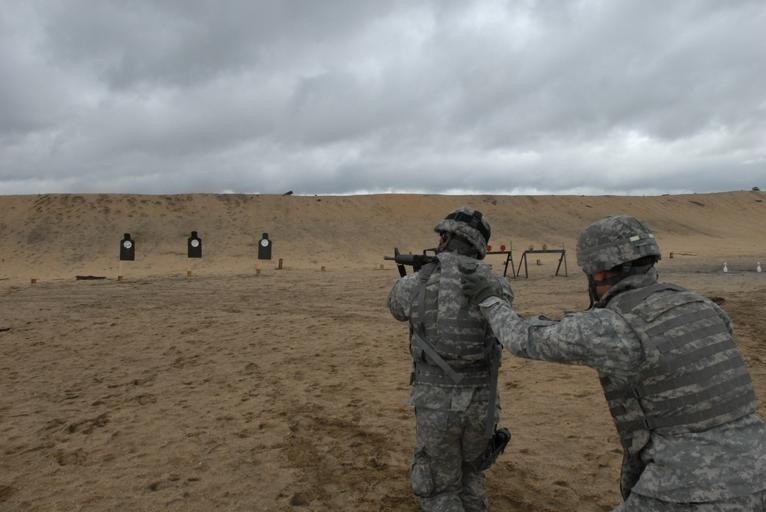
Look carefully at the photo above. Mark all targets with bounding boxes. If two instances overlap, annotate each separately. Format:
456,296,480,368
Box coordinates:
121,232,135,261
258,232,272,259
188,231,202,258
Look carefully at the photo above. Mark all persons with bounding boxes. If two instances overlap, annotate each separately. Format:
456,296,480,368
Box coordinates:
385,207,515,512
461,215,766,512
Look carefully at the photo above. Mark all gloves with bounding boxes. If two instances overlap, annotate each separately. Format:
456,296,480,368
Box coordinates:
461,272,500,305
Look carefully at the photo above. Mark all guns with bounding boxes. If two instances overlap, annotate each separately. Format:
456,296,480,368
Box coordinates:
383,246,437,277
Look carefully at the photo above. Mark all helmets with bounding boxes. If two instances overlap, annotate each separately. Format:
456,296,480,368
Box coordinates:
433,206,493,261
576,214,662,275
